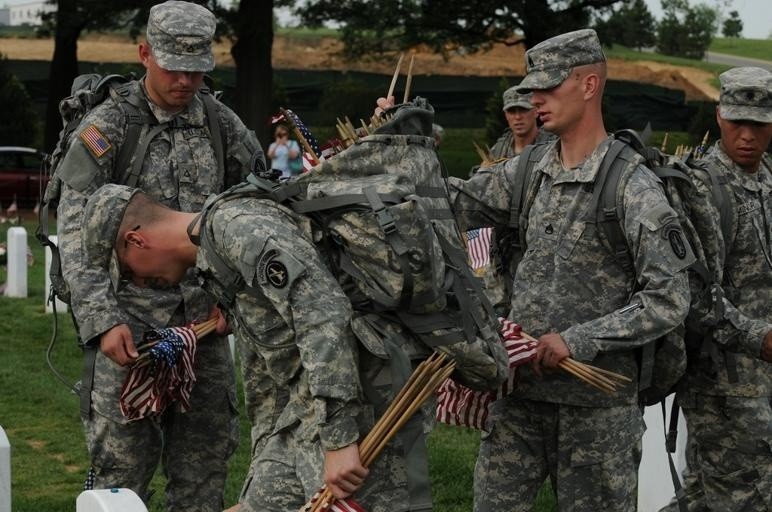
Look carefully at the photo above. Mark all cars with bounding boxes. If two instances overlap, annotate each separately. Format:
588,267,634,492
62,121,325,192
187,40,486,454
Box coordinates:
0,147,62,208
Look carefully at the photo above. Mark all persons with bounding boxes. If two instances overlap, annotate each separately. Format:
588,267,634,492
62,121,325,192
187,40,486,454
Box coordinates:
72,175,433,511
265,127,304,169
366,29,691,511
658,66,769,512
48,2,266,511
475,84,571,313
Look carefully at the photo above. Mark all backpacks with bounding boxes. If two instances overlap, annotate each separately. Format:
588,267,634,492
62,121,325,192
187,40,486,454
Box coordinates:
43,71,227,304
202,133,510,391
511,128,726,406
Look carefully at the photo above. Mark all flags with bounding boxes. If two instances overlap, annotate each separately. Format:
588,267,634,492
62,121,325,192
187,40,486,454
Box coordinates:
316,139,347,160
117,318,207,423
454,222,505,273
435,313,555,433
279,109,327,172
300,483,367,512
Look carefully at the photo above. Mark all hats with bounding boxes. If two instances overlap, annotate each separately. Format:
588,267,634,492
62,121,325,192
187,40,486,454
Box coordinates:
81,184,144,293
503,28,607,111
146,0,217,73
718,66,771,124
